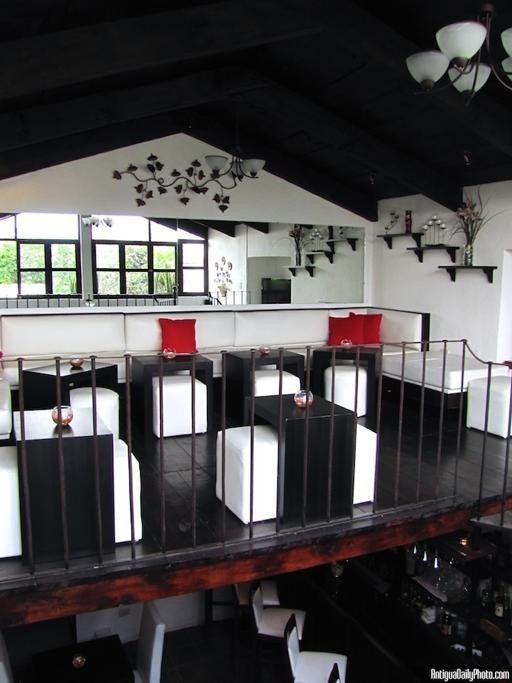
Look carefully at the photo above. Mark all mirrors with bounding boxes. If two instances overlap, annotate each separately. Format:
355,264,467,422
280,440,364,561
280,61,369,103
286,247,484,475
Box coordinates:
1,210,365,304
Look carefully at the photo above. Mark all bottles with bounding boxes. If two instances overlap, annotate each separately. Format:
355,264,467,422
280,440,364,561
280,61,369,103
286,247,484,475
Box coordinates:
408,542,511,635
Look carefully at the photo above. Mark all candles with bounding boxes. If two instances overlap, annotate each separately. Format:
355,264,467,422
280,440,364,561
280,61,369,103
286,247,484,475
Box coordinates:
309,228,323,239
423,214,445,229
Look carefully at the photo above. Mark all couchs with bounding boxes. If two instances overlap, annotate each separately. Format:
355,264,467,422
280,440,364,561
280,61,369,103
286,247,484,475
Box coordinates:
0,305,431,443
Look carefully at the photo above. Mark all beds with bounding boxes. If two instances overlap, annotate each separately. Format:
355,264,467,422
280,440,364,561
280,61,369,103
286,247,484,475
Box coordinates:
243,393,358,529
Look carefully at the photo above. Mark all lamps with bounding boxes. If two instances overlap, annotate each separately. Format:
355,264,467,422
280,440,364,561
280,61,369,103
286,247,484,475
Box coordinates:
405,3,512,110
204,93,267,182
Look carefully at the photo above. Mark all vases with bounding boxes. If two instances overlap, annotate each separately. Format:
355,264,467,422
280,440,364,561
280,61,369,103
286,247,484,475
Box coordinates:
296,249,300,266
464,244,472,266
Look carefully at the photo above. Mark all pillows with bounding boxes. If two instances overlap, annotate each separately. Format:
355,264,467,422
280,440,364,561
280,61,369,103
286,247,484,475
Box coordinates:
157,318,199,354
329,312,383,346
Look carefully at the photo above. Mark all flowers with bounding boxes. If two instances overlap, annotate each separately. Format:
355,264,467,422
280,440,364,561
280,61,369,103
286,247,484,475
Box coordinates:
448,182,506,247
286,224,311,252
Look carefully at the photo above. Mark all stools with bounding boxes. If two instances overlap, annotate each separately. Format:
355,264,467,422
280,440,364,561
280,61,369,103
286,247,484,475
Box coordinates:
324,364,366,418
250,369,301,397
466,375,511,438
215,422,377,525
152,375,208,438
1,439,144,559
69,388,119,439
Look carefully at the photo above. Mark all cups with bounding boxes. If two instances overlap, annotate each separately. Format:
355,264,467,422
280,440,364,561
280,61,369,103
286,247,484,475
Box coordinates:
293,389,314,408
162,347,177,359
259,344,271,355
70,356,84,368
70,654,87,671
340,338,353,350
51,404,75,425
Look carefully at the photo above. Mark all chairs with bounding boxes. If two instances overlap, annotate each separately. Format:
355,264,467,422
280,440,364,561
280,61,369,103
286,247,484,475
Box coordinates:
132,576,351,683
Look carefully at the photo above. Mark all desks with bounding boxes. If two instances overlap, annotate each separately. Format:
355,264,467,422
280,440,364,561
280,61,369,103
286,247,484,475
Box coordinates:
21,633,137,683
